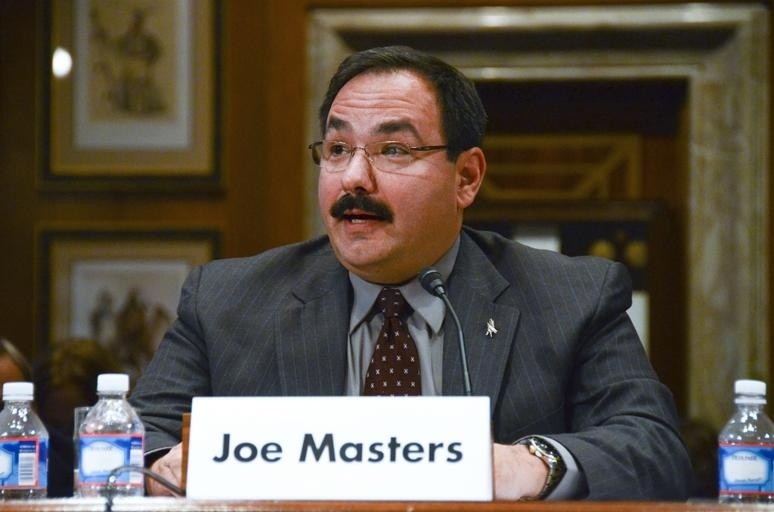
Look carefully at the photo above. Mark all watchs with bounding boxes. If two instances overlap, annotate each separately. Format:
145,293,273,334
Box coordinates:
518,437,566,502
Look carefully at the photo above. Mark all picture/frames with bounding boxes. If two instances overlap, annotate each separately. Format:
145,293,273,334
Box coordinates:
32,0,232,197
36,224,221,436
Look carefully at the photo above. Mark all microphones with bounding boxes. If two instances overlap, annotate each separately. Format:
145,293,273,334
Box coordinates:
419,265,473,397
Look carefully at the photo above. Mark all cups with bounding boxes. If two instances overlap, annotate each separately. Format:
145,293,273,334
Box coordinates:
69,404,92,511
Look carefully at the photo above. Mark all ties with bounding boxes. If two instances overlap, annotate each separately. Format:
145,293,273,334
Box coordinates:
361,289,423,397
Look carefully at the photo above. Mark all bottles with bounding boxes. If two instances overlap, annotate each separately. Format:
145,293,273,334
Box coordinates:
716,379,773,512
76,371,145,511
0,380,52,511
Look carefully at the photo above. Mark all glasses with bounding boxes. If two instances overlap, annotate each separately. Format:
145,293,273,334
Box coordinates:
308,138,446,174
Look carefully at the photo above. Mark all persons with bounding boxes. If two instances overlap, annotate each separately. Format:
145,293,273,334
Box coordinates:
0,337,75,499
125,43,699,504
